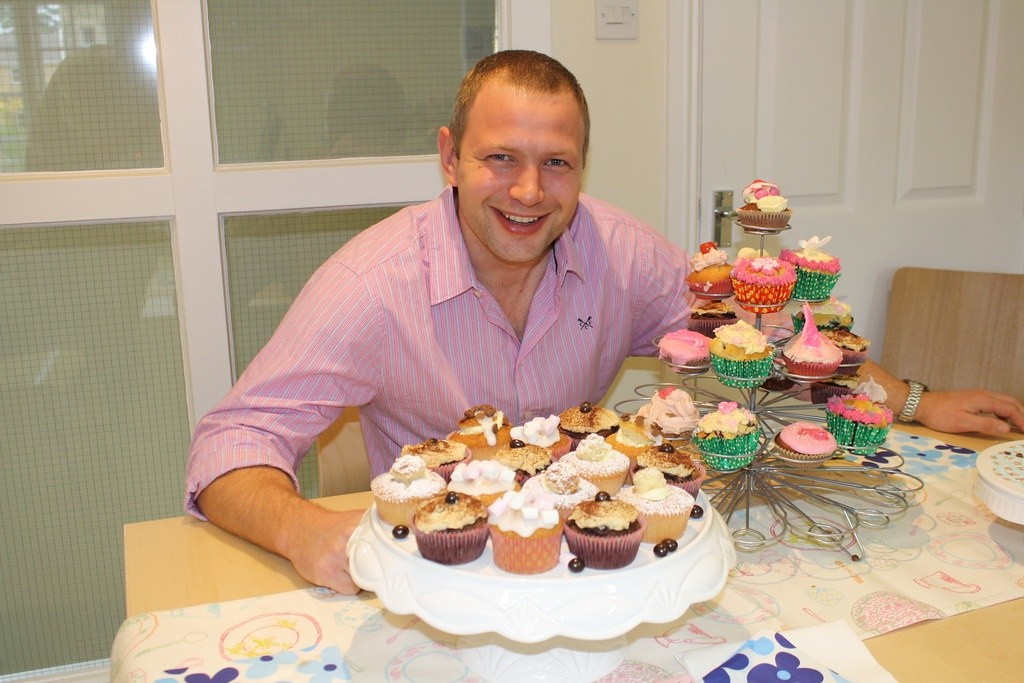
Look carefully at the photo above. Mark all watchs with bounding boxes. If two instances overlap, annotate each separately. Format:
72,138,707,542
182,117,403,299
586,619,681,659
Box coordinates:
898,379,929,424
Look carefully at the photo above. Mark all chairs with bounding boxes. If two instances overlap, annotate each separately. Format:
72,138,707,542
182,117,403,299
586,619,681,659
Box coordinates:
878,266,1024,409
318,400,373,499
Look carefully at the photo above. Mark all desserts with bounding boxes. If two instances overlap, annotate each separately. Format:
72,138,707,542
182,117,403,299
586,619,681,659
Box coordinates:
636,179,893,472
370,402,705,575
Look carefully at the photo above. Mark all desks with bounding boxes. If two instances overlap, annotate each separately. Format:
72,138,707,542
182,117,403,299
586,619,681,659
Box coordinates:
121,422,1024,682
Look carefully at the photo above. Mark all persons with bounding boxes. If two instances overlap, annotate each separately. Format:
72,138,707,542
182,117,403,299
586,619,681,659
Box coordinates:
183,50,1024,595
